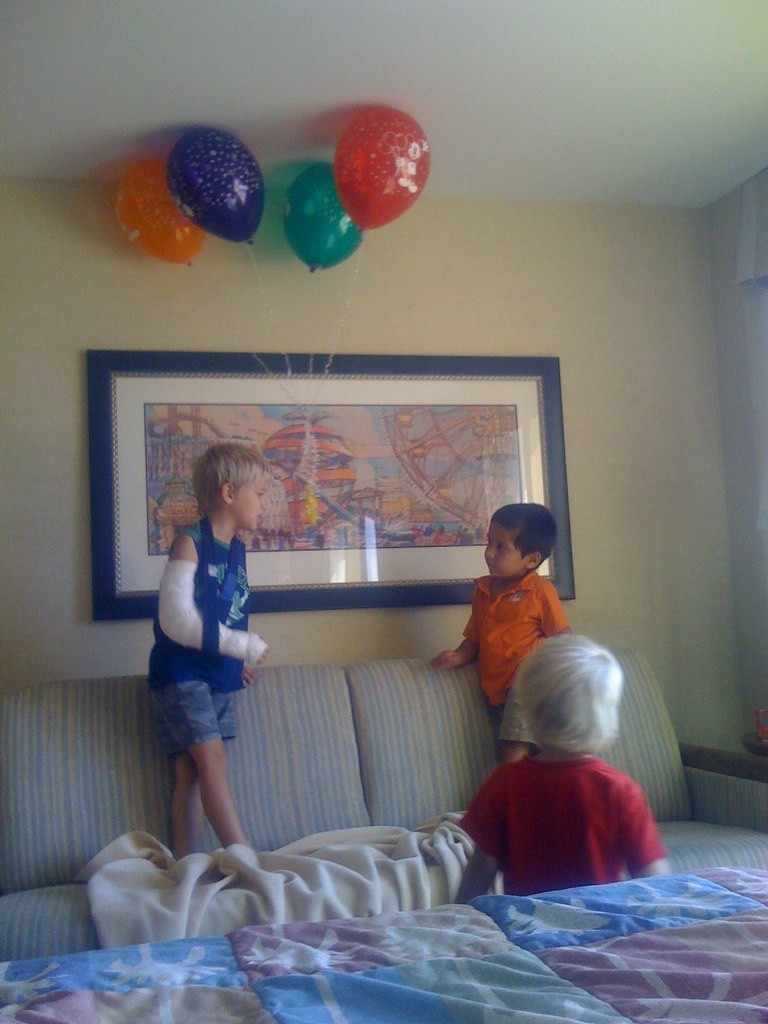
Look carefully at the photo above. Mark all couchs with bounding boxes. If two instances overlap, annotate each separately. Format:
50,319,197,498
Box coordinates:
0,645,768,962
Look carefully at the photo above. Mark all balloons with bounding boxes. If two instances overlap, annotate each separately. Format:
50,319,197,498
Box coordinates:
112,107,433,272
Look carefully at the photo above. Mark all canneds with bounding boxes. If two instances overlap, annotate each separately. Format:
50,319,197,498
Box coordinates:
755,708,768,741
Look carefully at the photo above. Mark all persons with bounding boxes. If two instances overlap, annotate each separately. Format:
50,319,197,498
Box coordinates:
453,633,676,905
431,503,571,764
148,443,274,857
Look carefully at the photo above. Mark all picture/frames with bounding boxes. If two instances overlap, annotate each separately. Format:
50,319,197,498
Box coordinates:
89,349,576,618
